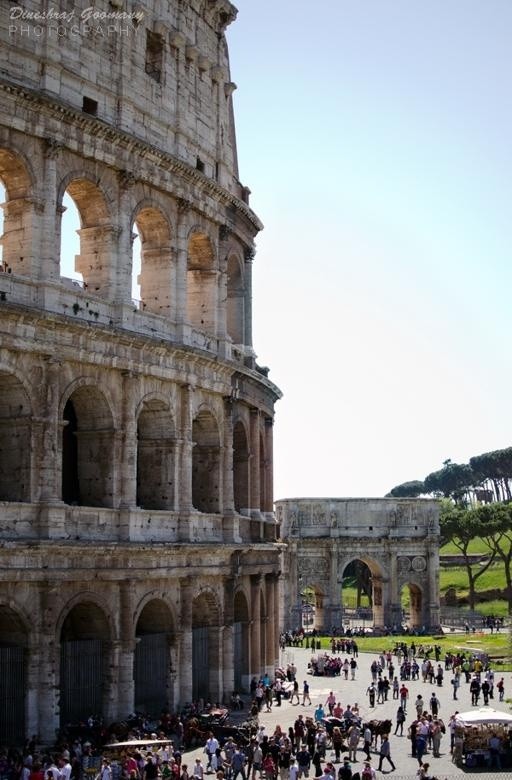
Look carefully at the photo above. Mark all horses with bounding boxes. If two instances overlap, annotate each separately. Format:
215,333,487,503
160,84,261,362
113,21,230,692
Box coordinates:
368,719,393,752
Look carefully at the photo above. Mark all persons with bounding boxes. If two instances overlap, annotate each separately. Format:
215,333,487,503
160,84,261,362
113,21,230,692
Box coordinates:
0,614,512,780
